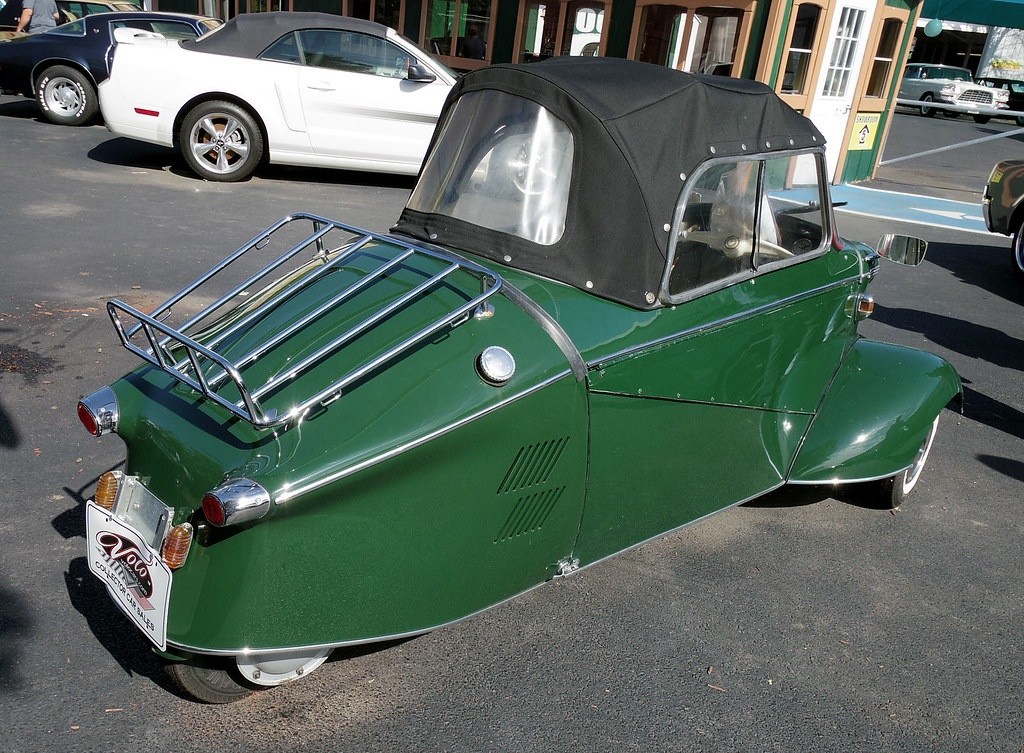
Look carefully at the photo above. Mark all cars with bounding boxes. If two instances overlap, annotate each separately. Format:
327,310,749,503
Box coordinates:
0,10,225,129
896,65,1013,125
91,10,567,206
0,1,163,33
81,51,971,706
982,159,1024,304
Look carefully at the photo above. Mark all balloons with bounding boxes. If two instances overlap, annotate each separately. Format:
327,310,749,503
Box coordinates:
924,19,942,37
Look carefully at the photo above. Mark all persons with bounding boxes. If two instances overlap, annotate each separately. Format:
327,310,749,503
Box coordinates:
17,0,59,34
458,23,483,59
908,67,927,78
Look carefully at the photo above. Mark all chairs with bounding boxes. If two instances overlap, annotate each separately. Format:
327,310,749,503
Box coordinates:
306,41,324,67
